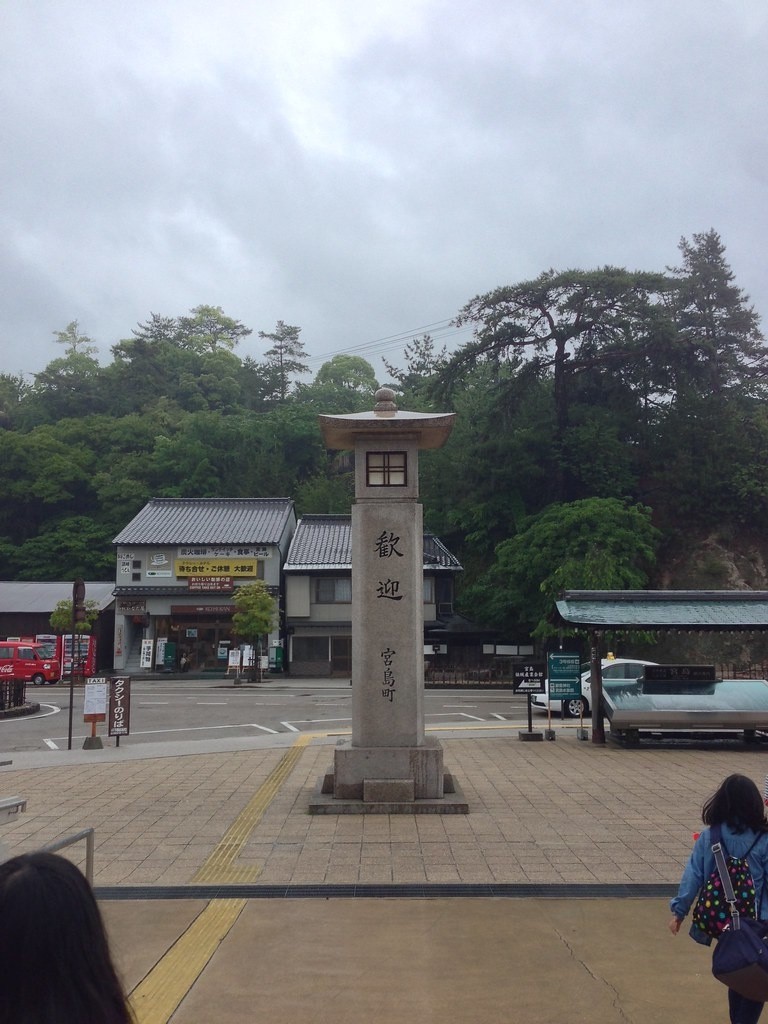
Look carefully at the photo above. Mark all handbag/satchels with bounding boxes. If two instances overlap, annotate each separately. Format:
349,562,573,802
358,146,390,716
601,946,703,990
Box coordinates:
712,917,768,1003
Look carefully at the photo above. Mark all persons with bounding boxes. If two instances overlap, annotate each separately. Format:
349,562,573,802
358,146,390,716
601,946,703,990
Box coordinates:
0,851,136,1024
668,773,768,1024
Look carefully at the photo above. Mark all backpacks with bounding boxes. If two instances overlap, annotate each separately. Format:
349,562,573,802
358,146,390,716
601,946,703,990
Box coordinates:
692,830,764,939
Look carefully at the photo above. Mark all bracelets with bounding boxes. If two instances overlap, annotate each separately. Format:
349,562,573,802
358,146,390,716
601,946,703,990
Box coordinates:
672,917,679,922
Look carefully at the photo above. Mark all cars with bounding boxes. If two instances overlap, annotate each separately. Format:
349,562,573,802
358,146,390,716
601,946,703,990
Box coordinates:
530,650,660,717
0,641,61,686
